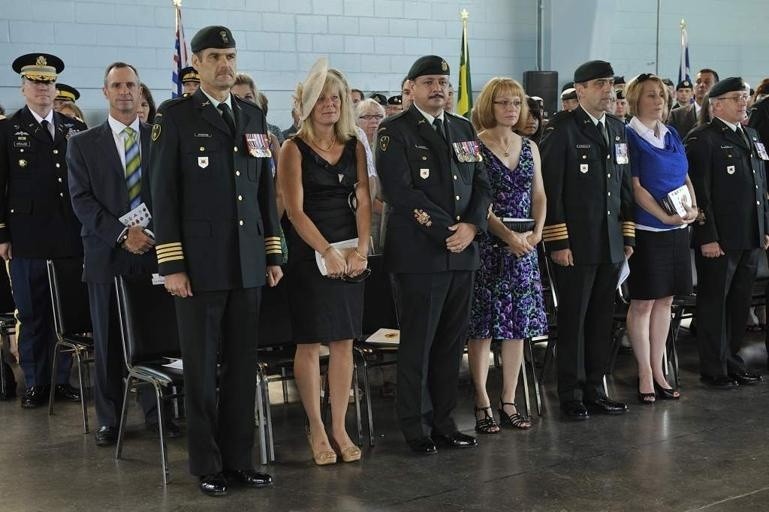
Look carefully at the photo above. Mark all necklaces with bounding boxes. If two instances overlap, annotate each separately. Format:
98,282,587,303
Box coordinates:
486,130,513,157
310,135,337,153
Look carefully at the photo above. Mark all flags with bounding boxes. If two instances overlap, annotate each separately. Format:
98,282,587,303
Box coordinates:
678,31,690,83
169,9,190,99
457,29,474,117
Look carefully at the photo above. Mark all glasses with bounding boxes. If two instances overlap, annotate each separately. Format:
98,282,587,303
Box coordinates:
713,93,751,104
633,72,654,88
492,99,525,108
345,267,373,284
714,95,749,102
357,113,387,122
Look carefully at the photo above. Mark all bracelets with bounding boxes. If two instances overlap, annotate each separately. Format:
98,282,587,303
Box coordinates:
321,245,333,259
353,248,367,261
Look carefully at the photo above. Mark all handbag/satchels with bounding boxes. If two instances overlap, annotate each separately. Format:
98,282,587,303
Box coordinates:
314,234,377,279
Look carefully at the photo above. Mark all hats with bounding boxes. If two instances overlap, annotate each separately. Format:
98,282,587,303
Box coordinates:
54,82,82,104
389,96,403,107
675,79,696,92
707,75,747,99
573,59,616,84
11,52,66,85
177,65,204,83
560,88,580,99
613,75,625,85
614,89,627,101
406,55,451,81
190,24,237,52
369,93,390,105
662,78,674,87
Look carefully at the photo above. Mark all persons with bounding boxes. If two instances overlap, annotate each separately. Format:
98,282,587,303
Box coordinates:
556,72,631,121
151,25,285,492
252,93,287,144
349,87,402,125
541,59,635,419
445,81,474,122
662,65,769,132
228,74,283,220
682,77,769,390
376,56,490,456
398,76,414,113
531,94,552,142
623,72,698,403
67,62,156,446
136,80,155,121
56,99,82,121
176,65,202,97
353,98,387,331
277,69,371,466
512,94,541,145
56,81,80,114
469,73,547,434
0,52,87,409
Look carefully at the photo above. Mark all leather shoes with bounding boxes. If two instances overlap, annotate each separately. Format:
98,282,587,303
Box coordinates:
700,371,741,391
198,470,230,497
407,433,440,455
585,394,630,417
559,398,592,422
729,364,764,385
20,383,49,409
52,382,82,401
228,467,274,489
94,422,126,449
436,426,480,450
144,418,183,440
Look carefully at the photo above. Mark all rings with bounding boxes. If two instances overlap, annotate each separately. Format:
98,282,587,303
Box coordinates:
171,293,175,295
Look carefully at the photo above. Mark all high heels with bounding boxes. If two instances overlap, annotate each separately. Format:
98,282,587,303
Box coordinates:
636,374,657,406
653,377,681,401
328,433,363,463
495,398,533,431
305,428,338,466
474,404,501,433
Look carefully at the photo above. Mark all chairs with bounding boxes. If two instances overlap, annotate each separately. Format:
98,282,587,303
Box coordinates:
669,293,698,388
527,239,611,416
115,274,266,488
359,258,400,447
602,288,667,396
256,327,364,462
48,258,94,435
461,338,531,421
0,256,17,399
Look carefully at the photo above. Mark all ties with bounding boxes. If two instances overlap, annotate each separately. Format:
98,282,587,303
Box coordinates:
40,119,54,147
596,119,610,150
736,125,752,151
123,125,144,212
433,118,450,145
216,101,238,139
